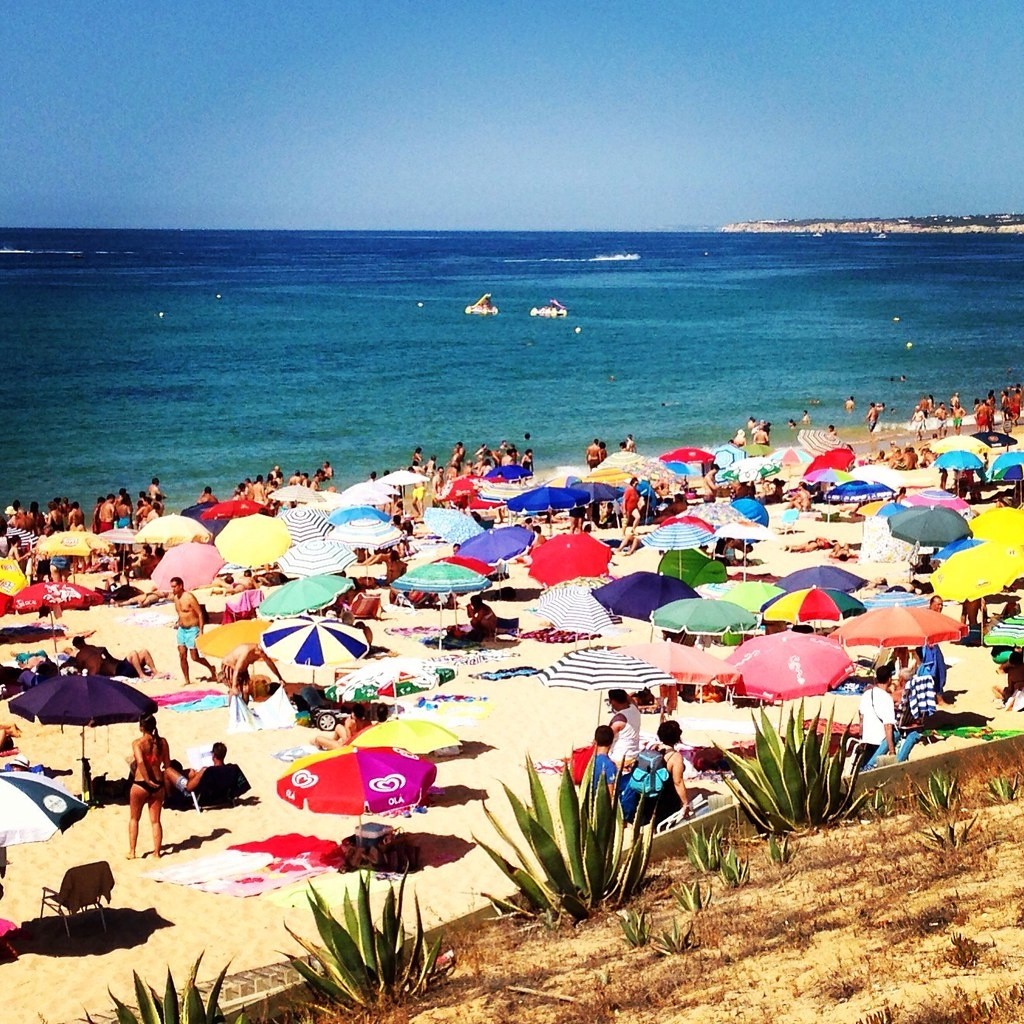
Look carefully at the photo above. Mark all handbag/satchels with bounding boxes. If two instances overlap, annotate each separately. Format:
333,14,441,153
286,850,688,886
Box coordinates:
617,769,656,825
571,743,597,785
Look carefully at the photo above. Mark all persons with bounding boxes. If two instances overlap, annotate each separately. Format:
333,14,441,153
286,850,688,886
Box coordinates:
856,600,1024,775
586,658,726,835
0,703,390,906
0,577,400,708
0,439,545,657
568,374,1024,634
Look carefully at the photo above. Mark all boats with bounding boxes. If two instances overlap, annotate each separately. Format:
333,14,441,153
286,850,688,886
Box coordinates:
529,299,567,318
465,293,499,316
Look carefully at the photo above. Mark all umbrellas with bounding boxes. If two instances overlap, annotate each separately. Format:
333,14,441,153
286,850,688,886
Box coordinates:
0,772,92,848
0,468,431,688
825,432,1024,649
524,566,968,748
280,656,464,844
390,429,855,605
8,673,158,790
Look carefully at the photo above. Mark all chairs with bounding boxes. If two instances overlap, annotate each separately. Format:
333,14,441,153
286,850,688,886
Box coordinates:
340,593,387,622
493,617,521,642
693,682,766,707
38,861,115,938
774,509,799,536
630,691,659,714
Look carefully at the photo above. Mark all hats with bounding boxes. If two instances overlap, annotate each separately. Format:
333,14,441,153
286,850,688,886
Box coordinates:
9,753,29,769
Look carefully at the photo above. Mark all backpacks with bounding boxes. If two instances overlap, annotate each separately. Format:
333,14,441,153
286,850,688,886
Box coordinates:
628,745,677,797
